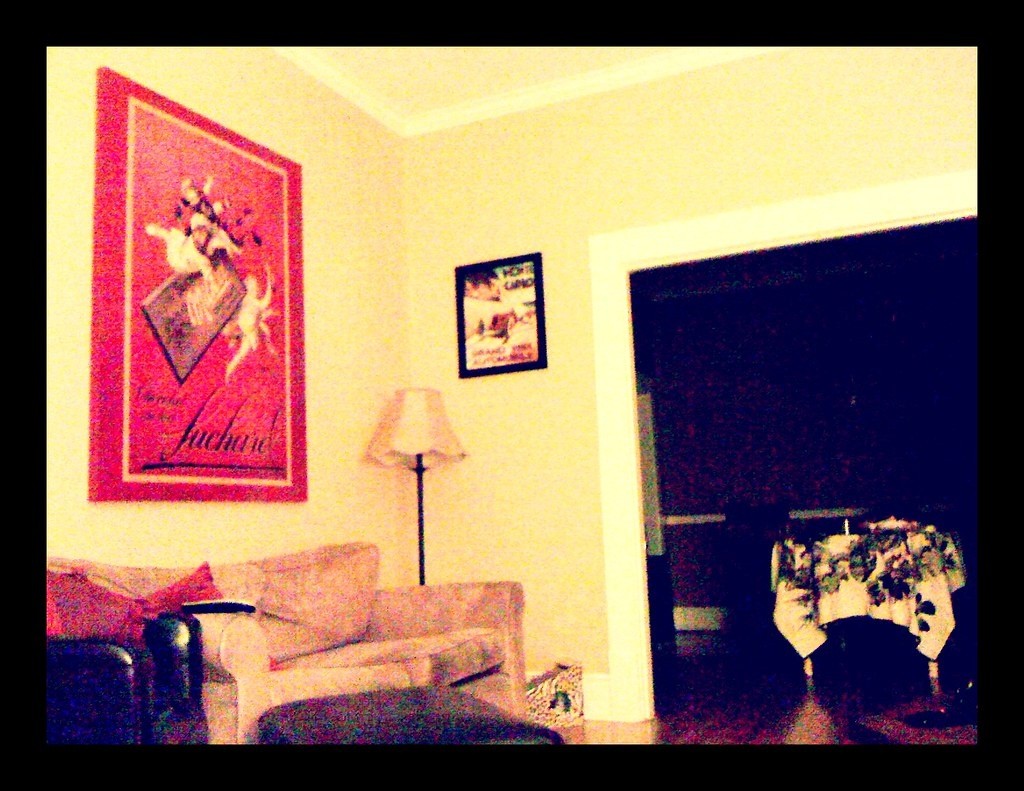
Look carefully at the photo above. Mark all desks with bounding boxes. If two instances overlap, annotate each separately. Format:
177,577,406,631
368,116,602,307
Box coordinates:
769,529,968,680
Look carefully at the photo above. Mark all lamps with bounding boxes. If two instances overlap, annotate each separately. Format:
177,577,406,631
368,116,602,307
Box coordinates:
366,386,470,585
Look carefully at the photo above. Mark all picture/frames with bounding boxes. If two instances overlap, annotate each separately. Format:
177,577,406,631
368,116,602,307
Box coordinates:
455,252,547,380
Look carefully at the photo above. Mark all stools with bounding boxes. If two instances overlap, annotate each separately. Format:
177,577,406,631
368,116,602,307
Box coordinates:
258,683,566,745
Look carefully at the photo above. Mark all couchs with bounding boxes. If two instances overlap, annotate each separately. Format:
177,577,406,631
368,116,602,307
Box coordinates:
47,540,528,745
